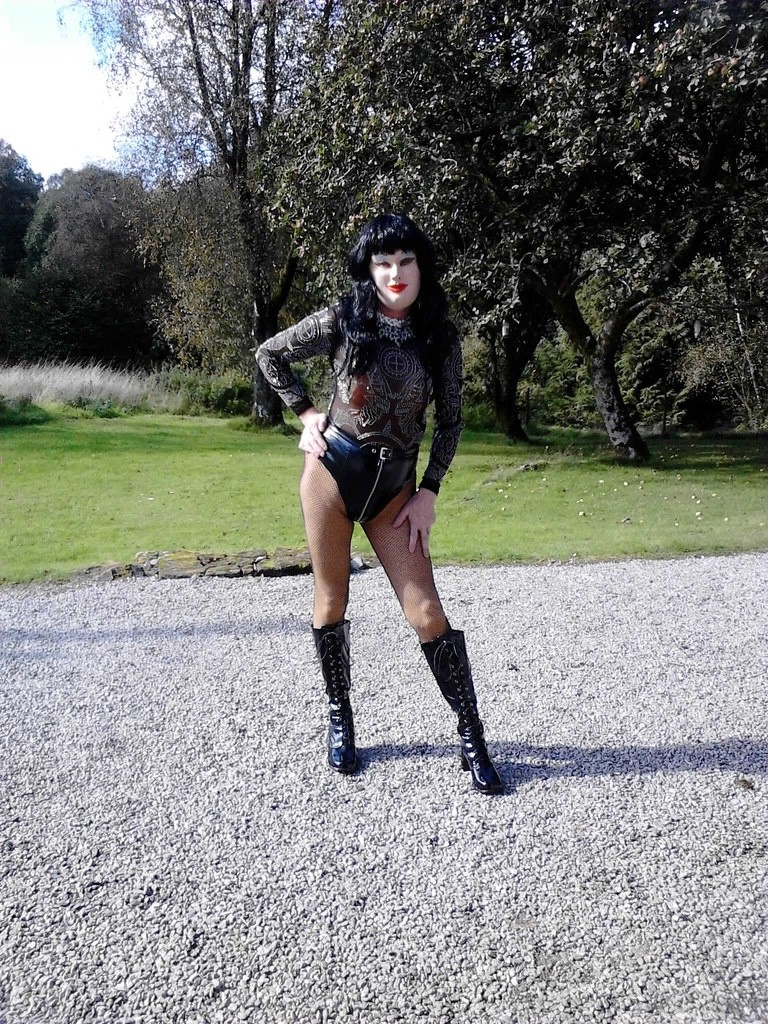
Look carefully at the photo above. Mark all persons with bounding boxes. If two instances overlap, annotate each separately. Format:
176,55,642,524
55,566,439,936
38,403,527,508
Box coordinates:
252,214,508,795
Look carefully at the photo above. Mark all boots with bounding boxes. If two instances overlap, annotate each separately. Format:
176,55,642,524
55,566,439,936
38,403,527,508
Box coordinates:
312,620,358,773
421,630,502,793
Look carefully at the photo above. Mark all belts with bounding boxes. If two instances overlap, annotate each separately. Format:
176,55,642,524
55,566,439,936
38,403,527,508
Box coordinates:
331,425,419,460
